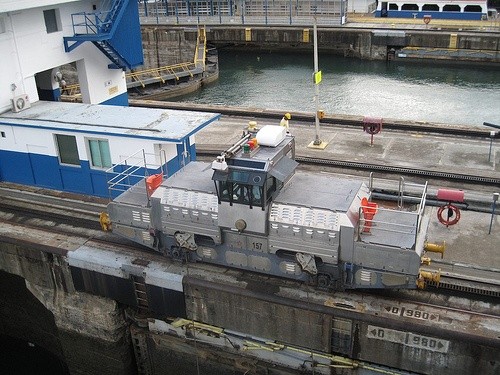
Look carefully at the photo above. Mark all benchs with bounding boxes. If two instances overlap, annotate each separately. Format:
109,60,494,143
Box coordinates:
361,197,378,235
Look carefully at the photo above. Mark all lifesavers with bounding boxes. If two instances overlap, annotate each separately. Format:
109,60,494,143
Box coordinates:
438,205,461,226
365,122,380,134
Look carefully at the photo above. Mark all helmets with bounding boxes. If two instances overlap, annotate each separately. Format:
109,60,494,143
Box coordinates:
285,112,291,120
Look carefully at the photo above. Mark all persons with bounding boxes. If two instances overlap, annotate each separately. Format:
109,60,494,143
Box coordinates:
280,113,291,129
61,78,70,95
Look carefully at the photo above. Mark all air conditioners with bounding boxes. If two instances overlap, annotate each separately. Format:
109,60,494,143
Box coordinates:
10,94,31,113
